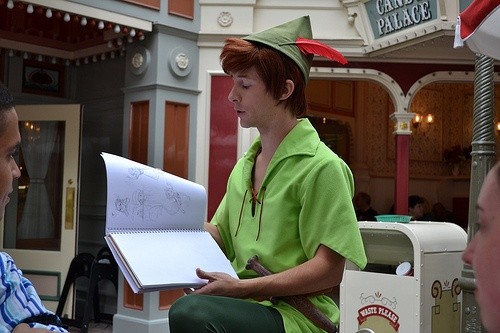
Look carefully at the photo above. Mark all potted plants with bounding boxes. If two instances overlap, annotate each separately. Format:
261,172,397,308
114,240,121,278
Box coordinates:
443,145,472,177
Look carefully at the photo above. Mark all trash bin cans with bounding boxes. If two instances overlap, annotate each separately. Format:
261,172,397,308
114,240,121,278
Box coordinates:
340,219,466,333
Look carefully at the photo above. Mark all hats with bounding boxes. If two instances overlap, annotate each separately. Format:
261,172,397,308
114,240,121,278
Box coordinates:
241,15,348,89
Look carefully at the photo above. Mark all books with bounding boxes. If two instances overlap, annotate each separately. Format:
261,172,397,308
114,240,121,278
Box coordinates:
101,152,239,294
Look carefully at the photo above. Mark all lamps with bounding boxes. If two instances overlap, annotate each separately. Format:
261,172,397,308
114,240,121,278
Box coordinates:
413,114,434,136
25,121,41,142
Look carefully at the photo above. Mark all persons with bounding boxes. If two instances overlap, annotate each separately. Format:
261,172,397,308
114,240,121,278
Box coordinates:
168,15,368,333
351,190,462,226
0,82,71,333
462,159,500,333
